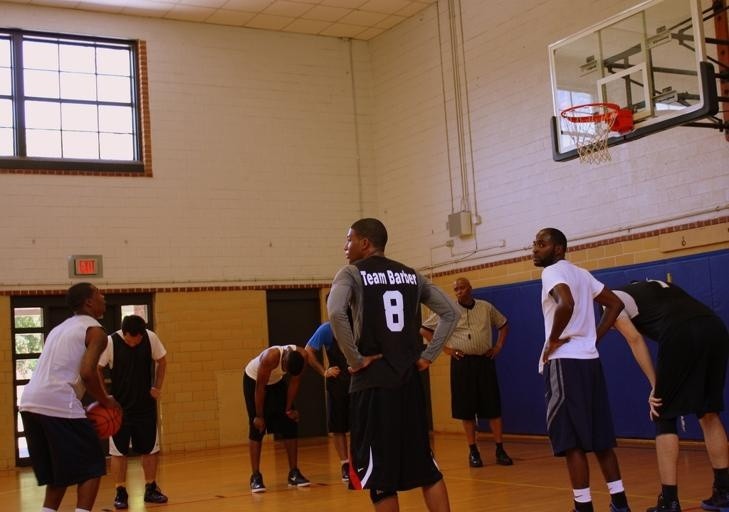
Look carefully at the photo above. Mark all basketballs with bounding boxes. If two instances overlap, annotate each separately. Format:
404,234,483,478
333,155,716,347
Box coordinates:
86,401,123,440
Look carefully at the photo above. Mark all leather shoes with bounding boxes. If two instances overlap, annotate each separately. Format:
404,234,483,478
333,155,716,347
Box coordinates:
470,449,482,466
495,448,513,465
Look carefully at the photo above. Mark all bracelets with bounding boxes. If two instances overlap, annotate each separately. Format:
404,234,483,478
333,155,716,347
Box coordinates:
285,408,293,416
150,386,161,393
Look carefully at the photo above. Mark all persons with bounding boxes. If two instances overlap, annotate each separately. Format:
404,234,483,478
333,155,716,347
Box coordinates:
306,294,356,481
97,315,168,508
327,218,460,512
421,278,514,467
531,227,632,512
243,344,312,493
601,280,728,512
18,282,121,512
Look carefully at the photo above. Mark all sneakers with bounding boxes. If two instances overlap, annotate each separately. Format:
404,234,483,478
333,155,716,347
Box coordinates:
249,471,266,493
608,502,631,512
288,467,312,486
144,480,168,503
646,494,681,512
702,485,729,512
341,463,350,482
114,485,128,508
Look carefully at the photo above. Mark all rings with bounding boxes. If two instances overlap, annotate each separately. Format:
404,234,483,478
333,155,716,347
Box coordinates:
455,351,458,356
